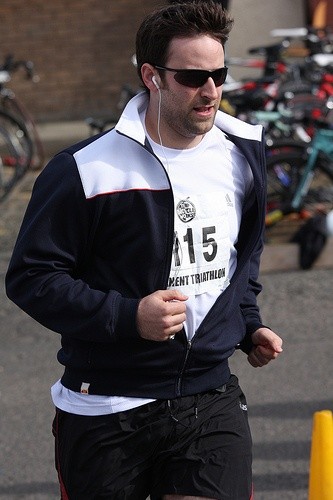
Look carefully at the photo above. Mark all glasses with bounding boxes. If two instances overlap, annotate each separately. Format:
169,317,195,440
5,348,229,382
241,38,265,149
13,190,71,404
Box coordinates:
154,66,228,87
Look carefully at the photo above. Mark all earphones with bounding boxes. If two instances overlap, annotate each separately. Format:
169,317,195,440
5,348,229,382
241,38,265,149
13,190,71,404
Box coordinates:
152,75,160,89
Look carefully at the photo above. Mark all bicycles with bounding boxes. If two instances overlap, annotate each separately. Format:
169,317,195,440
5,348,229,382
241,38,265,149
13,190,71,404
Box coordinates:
0,22,333,271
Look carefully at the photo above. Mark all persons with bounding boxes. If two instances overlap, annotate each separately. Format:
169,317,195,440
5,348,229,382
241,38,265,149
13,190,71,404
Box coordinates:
5,1,285,499
